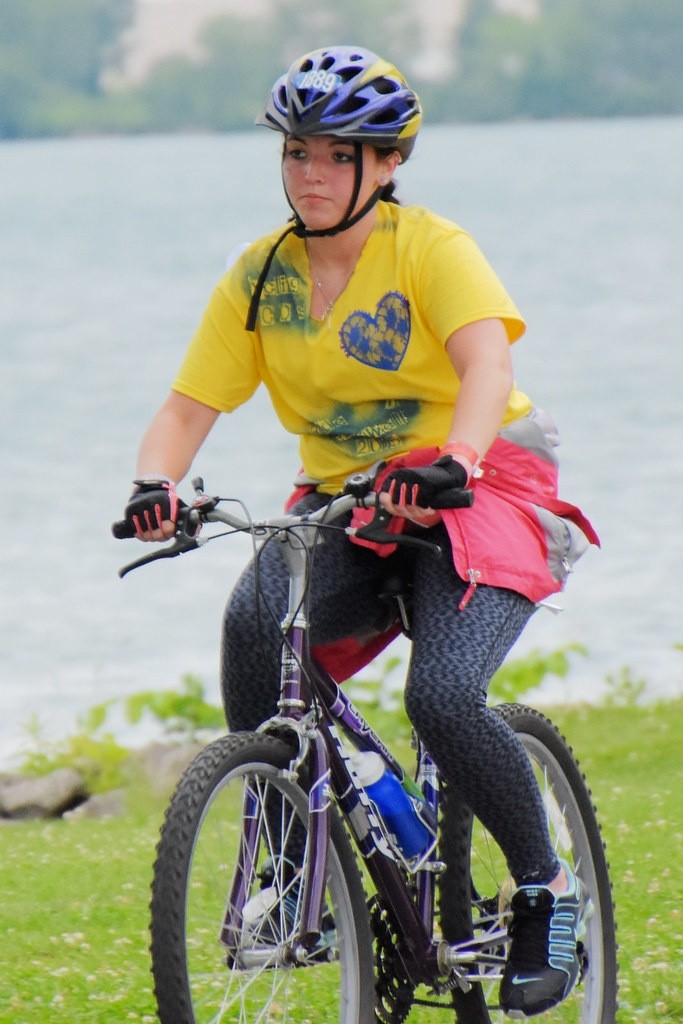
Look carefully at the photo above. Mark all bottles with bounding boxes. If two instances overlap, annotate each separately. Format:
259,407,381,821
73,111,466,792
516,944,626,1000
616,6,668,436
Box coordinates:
348,750,432,858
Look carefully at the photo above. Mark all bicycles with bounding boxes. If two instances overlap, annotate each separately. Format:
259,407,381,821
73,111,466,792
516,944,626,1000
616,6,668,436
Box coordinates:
110,458,619,1023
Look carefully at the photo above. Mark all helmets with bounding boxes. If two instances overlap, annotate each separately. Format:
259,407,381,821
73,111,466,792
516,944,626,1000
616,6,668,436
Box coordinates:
253,44,423,168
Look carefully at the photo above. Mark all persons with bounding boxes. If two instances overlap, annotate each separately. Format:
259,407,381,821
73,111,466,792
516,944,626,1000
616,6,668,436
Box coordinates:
124,46,594,1016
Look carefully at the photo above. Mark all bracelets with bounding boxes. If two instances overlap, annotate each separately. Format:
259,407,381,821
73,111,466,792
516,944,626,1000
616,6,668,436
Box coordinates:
439,441,479,467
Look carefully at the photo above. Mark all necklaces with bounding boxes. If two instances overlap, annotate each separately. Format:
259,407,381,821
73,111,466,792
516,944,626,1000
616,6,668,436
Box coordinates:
308,264,334,309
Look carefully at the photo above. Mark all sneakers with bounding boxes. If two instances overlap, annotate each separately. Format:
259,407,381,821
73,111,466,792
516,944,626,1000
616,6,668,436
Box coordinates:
497,859,598,1020
236,854,338,959
